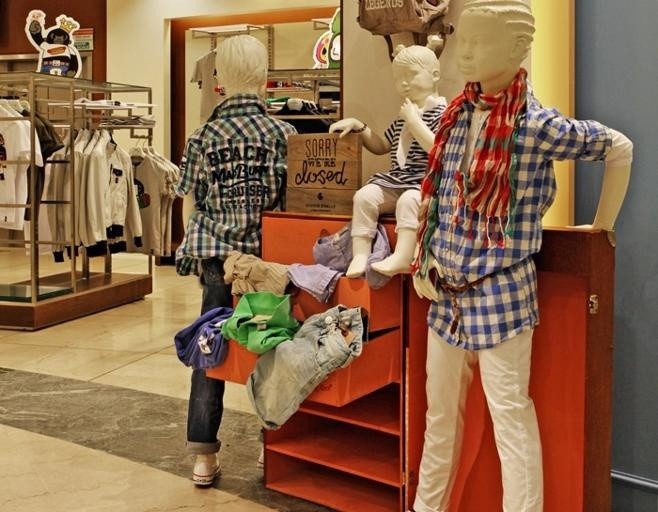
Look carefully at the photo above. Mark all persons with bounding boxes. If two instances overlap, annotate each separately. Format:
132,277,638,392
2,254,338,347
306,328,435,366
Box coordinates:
327,46,453,281
156,36,294,489
410,0,634,512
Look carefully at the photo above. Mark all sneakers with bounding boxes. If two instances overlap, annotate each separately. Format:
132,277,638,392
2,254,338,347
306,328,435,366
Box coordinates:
256,446,266,472
191,453,223,487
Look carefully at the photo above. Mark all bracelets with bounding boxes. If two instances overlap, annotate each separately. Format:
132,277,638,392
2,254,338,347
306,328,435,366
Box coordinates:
352,123,370,132
